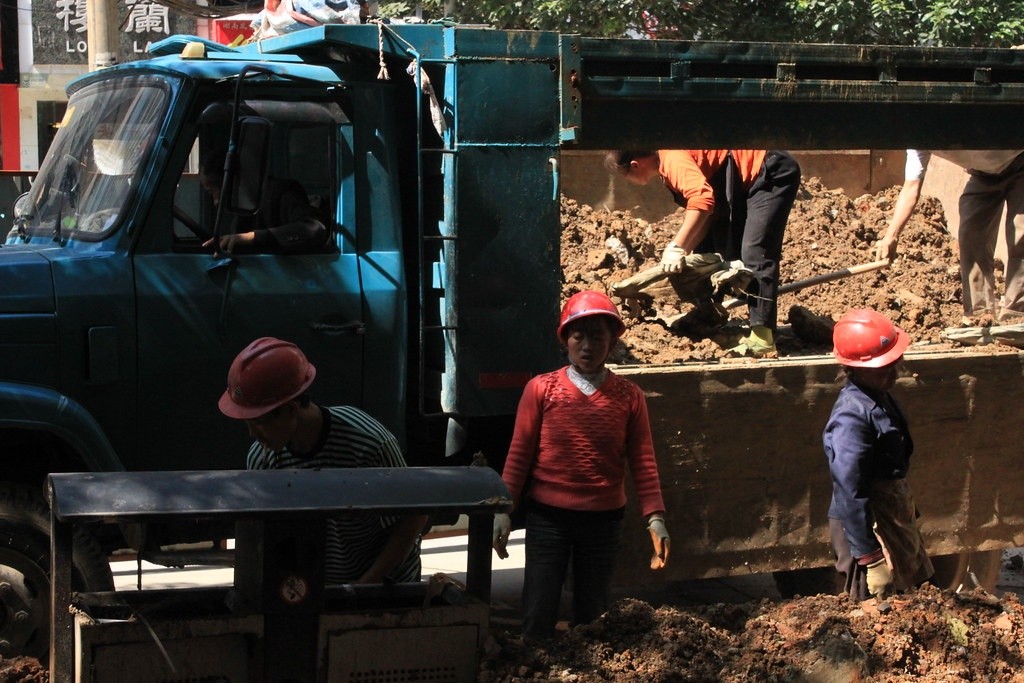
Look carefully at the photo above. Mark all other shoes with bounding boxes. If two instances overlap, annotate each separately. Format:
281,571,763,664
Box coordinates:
666,311,727,336
727,339,777,358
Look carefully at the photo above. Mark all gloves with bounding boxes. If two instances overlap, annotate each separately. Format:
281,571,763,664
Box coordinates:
493,513,511,560
648,517,670,569
866,558,897,599
660,245,686,274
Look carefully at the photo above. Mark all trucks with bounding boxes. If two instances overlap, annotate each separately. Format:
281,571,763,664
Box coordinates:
1,21,1024,658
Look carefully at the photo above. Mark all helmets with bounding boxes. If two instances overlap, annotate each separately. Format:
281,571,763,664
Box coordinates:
832,309,909,369
218,337,316,420
557,290,626,347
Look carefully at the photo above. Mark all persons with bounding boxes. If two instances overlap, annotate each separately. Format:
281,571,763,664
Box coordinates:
822,309,936,604
201,146,327,260
492,291,670,638
218,337,421,584
603,150,801,358
876,148,1024,316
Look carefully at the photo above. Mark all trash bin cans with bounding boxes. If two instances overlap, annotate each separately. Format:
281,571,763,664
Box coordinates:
47,465,514,683
